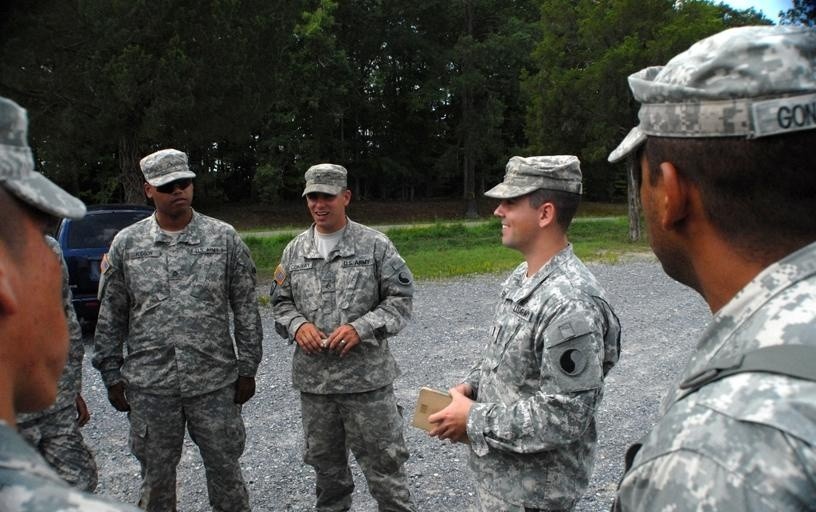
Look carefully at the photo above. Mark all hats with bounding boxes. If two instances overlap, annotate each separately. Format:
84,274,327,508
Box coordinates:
605,22,815,162
483,154,587,199
301,161,347,197
139,148,198,188
0,96,89,228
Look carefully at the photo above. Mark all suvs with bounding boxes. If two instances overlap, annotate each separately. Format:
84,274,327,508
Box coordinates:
51,205,158,323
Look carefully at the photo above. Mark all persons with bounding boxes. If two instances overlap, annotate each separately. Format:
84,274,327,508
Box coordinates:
603,26,816,512
269,163,414,512
0,96,140,511
94,146,263,511
16,233,98,492
428,156,622,511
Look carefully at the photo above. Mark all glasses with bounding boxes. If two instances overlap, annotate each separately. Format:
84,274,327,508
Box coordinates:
156,179,192,194
